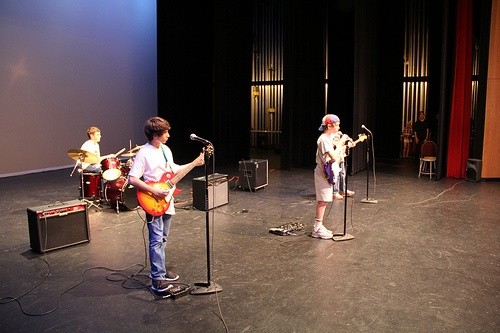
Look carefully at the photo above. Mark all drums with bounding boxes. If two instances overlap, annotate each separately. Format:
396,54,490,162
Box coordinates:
118,158,134,177
103,175,144,210
79,170,102,201
99,154,122,181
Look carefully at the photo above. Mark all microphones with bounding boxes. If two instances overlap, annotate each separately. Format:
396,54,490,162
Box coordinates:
337,130,353,143
190,133,211,144
361,124,372,134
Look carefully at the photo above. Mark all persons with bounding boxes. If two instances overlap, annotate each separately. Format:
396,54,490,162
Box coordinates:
413,111,429,170
127,117,204,292
80,127,116,173
312,114,345,239
332,130,356,200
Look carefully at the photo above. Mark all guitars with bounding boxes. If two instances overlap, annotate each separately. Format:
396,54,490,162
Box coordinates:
323,140,355,185
135,143,212,218
344,133,368,148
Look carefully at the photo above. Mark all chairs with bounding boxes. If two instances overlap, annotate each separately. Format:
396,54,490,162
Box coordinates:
418,141,437,179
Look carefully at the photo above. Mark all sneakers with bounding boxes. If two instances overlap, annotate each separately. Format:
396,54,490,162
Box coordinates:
311,225,333,240
150,279,173,292
149,272,179,280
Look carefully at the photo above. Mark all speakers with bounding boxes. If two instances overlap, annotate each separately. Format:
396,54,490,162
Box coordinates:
191,172,229,211
465,158,482,181
26,199,90,254
347,141,368,173
239,158,269,191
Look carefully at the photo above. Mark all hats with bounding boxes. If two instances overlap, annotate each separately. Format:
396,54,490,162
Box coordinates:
318,114,340,131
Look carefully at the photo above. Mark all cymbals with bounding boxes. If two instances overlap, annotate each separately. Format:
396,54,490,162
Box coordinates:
127,144,145,152
65,149,100,165
121,153,136,157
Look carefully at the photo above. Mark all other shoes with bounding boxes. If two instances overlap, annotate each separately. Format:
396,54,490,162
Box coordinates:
340,190,355,196
333,193,343,200
